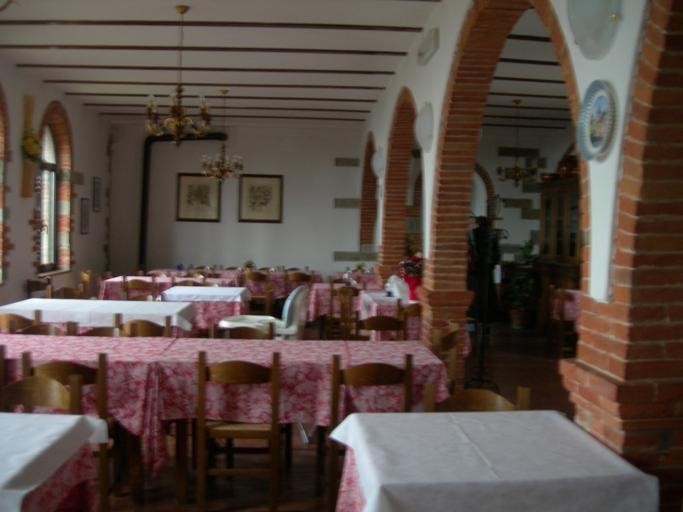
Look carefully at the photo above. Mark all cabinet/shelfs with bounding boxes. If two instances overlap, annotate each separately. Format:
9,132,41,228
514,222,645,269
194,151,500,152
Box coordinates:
531,175,581,355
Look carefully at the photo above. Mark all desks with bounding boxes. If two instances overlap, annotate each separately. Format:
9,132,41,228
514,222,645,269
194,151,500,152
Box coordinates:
324,409,661,511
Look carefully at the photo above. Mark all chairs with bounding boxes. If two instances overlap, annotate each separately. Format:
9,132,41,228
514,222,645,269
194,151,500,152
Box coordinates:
639,466,682,512
422,381,532,411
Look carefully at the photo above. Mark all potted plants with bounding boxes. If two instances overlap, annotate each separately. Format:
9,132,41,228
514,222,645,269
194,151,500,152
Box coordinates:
506,270,537,332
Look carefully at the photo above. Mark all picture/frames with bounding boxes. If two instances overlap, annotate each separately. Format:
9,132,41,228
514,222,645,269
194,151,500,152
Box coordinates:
176,172,221,222
80,197,90,234
237,174,284,223
93,176,102,212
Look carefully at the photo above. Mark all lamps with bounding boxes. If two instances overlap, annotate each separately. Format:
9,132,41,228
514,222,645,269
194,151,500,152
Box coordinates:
567,0,623,60
414,101,434,153
369,144,385,178
143,6,213,148
496,99,537,188
199,90,245,186
